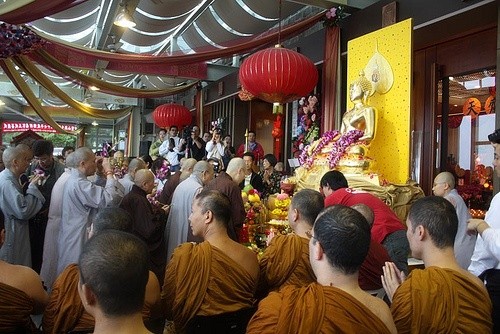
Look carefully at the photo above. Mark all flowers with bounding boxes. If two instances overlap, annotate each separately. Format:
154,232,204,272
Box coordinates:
148,191,161,206
242,191,292,248
32,168,46,177
323,6,345,26
292,94,319,158
155,165,170,180
101,143,112,157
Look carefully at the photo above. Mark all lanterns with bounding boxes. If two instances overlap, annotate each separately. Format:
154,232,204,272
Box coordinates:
153,102,192,130
239,45,318,160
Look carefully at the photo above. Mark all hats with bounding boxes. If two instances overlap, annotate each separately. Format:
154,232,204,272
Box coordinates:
207,157,220,164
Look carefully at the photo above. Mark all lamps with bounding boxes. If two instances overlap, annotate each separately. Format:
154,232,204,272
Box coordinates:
201,80,208,91
114,0,137,28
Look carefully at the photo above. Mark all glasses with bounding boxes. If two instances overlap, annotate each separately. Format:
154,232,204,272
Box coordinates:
35,158,48,164
306,228,318,241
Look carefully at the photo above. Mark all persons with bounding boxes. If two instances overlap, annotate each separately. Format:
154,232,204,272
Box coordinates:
334,76,378,141
0,125,500,334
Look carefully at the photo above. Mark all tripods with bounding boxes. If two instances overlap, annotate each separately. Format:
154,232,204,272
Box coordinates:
183,136,194,159
